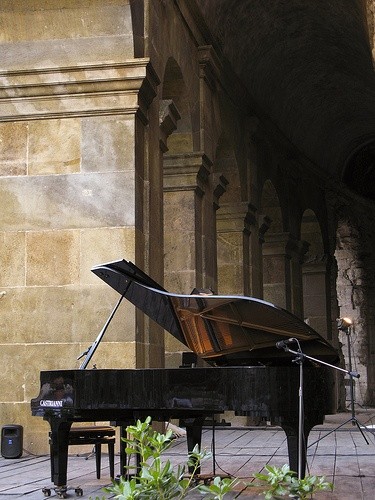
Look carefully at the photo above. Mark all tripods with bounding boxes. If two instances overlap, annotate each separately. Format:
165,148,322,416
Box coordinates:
307,329,375,448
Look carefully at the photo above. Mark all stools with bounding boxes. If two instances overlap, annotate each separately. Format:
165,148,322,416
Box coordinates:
49,426,116,482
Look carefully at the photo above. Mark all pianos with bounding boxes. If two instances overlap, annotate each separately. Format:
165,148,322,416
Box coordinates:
30,257,346,486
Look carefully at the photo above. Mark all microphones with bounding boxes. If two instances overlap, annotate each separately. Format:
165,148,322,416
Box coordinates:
276,338,296,348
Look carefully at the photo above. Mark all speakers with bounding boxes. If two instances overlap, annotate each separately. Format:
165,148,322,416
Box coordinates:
1,425,24,459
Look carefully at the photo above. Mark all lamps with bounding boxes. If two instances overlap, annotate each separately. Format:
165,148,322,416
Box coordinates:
337,317,358,426
